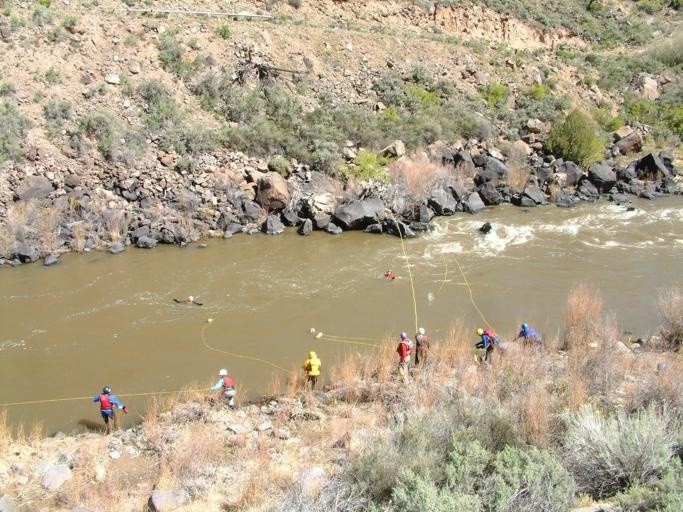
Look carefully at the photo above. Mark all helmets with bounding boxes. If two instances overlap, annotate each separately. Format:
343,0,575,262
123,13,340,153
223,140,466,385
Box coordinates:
520,322,528,329
218,369,227,375
475,328,483,336
102,386,111,394
418,327,425,335
400,332,407,339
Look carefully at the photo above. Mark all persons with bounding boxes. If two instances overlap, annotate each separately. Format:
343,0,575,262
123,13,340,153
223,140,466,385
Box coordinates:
172,295,203,307
90,385,129,435
473,327,501,362
395,331,413,374
208,367,236,409
413,328,431,367
511,322,542,349
383,269,396,280
302,351,322,390
